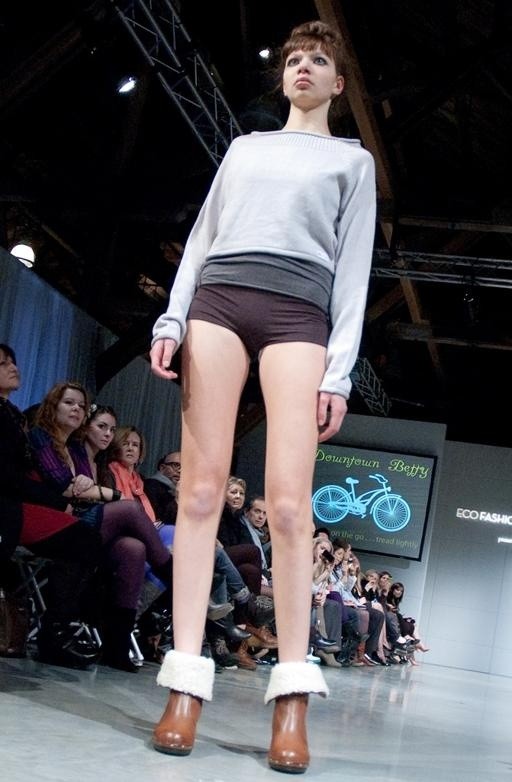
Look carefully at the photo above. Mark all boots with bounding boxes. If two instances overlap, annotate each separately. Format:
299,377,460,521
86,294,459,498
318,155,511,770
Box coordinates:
315,648,343,669
103,603,143,674
149,650,217,758
237,589,275,629
150,553,226,595
261,659,331,776
314,606,344,654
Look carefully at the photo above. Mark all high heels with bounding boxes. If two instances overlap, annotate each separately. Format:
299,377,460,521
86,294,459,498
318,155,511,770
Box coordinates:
383,642,396,651
415,643,430,652
407,655,420,666
371,651,391,667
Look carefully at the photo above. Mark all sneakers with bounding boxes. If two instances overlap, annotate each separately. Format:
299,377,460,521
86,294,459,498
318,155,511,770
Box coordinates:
209,637,240,668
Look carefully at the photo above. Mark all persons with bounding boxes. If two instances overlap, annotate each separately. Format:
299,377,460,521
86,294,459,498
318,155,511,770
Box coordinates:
142,18,382,774
312,524,431,670
1,342,279,672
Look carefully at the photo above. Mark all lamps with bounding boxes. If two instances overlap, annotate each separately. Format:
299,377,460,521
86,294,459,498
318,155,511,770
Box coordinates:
9,239,40,271
113,73,137,96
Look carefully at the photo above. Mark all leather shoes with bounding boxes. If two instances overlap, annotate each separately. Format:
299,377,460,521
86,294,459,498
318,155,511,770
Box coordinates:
410,638,421,645
223,623,251,642
233,644,258,671
313,632,337,649
206,598,233,622
243,624,281,649
392,636,411,647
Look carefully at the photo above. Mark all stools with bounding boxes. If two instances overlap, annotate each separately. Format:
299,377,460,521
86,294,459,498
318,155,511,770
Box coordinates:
11,552,49,653
